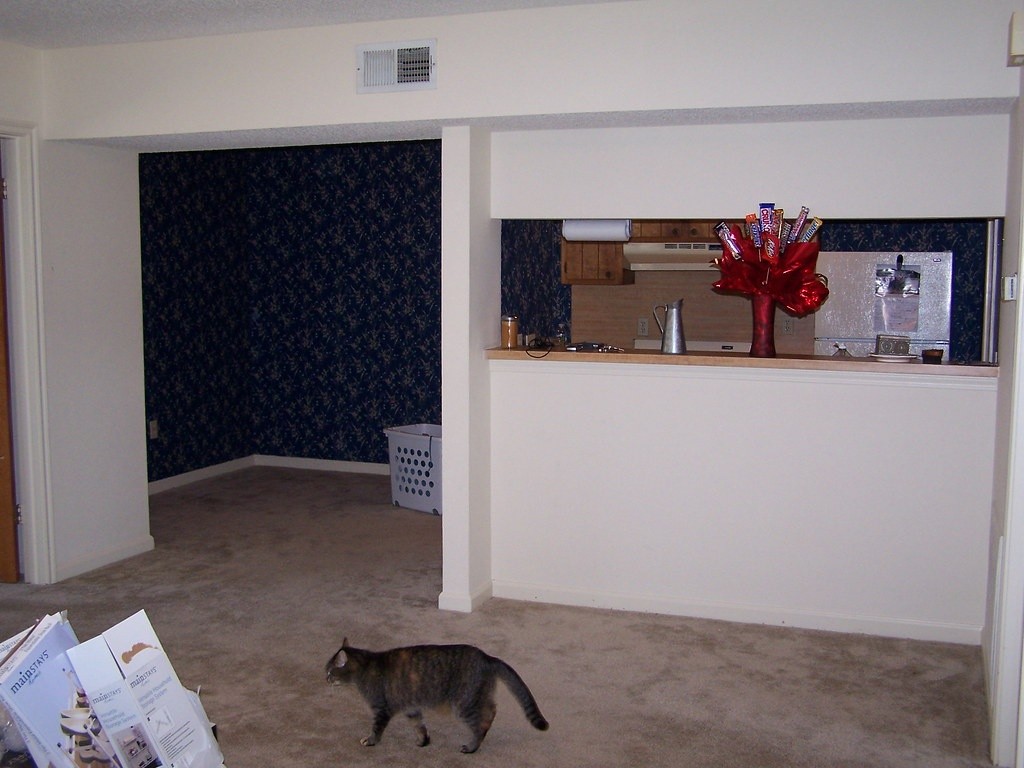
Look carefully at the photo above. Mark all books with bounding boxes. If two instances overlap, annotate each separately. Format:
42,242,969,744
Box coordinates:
0,613,124,768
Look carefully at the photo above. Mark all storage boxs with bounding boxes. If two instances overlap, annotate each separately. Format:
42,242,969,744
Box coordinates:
383,422,443,516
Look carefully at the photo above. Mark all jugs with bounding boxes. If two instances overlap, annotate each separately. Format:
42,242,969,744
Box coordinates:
652,297,687,355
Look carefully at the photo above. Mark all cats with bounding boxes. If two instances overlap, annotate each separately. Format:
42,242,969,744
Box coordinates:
325,636,550,753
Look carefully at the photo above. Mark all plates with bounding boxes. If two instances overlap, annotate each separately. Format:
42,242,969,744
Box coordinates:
869,352,917,364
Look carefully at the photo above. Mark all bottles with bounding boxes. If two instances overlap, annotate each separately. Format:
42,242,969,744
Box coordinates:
501,314,518,349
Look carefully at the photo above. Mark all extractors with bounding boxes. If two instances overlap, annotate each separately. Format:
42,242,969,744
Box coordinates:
623,242,725,270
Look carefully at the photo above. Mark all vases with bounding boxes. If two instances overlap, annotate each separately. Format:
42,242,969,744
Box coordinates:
748,294,776,357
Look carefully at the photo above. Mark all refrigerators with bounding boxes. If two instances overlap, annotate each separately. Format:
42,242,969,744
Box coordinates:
814,251,955,366
